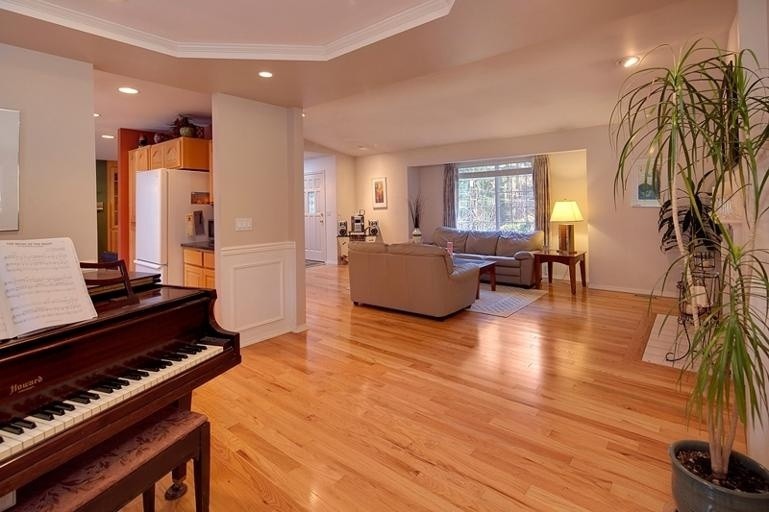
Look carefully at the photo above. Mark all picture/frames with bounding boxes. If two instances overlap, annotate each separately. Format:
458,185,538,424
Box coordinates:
629,156,669,208
0,108,21,232
372,176,387,209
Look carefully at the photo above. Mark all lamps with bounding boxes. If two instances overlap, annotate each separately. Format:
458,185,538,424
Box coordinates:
550,198,584,254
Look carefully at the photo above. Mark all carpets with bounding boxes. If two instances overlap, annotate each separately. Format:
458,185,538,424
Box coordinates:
642,314,736,378
465,282,549,318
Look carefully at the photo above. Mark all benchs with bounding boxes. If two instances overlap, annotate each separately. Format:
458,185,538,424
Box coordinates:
20,412,209,512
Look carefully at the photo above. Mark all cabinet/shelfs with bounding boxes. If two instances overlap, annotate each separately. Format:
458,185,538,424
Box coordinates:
129,137,214,288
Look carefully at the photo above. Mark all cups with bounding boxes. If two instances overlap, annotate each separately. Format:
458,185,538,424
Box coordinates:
447,242,453,255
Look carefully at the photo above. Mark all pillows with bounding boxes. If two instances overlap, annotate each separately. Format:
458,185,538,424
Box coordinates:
434,226,544,256
348,241,453,273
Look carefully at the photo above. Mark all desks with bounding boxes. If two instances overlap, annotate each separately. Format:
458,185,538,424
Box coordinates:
533,249,587,295
452,257,496,299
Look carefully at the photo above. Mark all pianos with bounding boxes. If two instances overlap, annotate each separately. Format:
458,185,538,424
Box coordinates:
2,257,241,502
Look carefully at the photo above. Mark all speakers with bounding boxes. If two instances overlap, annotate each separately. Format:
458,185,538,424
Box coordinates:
338,221,347,236
369,220,379,235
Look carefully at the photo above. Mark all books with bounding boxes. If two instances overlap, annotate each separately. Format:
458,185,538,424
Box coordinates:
0,238,99,342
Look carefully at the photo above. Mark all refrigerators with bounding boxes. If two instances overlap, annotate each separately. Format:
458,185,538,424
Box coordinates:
134,166,214,286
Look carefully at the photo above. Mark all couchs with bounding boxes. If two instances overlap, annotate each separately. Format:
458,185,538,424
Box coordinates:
348,241,480,321
430,226,545,289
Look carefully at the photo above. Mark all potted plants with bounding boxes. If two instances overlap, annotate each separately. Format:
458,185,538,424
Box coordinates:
610,35,769,512
